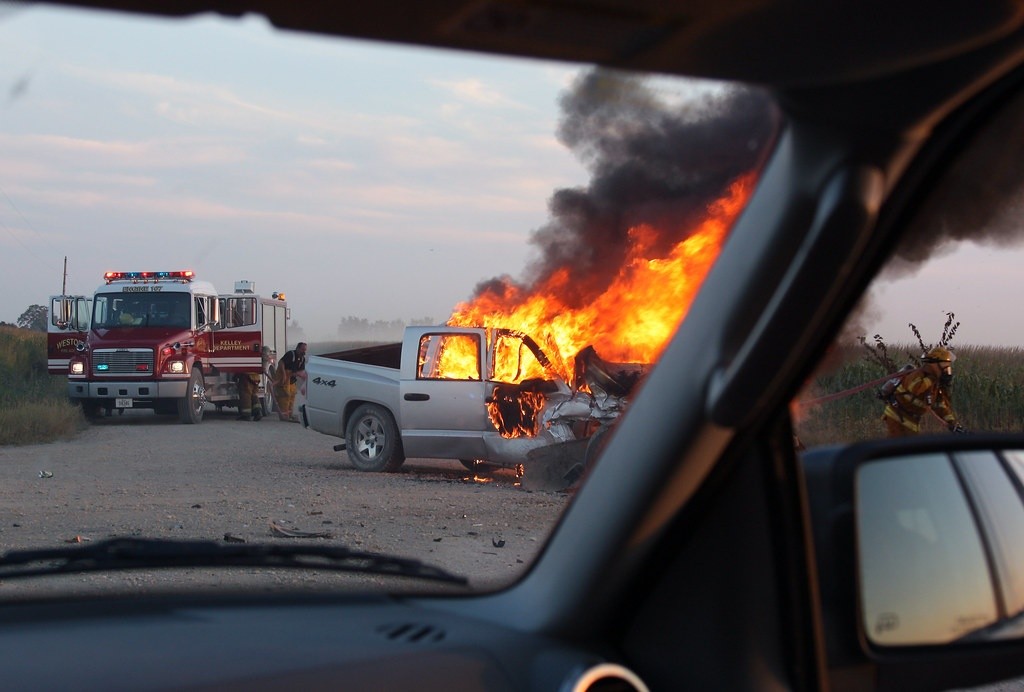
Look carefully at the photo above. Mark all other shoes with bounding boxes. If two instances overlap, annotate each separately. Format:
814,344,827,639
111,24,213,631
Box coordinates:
236,415,252,422
281,416,300,423
253,410,263,420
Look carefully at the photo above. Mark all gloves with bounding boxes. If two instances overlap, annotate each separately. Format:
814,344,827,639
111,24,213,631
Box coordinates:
948,422,964,432
927,373,936,383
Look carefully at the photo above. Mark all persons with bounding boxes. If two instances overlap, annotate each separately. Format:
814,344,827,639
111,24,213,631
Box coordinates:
274,342,307,421
880,348,962,438
238,373,264,421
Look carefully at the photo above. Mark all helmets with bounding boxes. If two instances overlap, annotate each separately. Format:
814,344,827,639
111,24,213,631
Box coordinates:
928,347,951,369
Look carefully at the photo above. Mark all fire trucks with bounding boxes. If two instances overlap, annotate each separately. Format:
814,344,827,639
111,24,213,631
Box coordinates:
47,271,305,426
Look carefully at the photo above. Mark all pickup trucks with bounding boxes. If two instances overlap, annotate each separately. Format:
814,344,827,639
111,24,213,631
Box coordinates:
298,325,655,493
